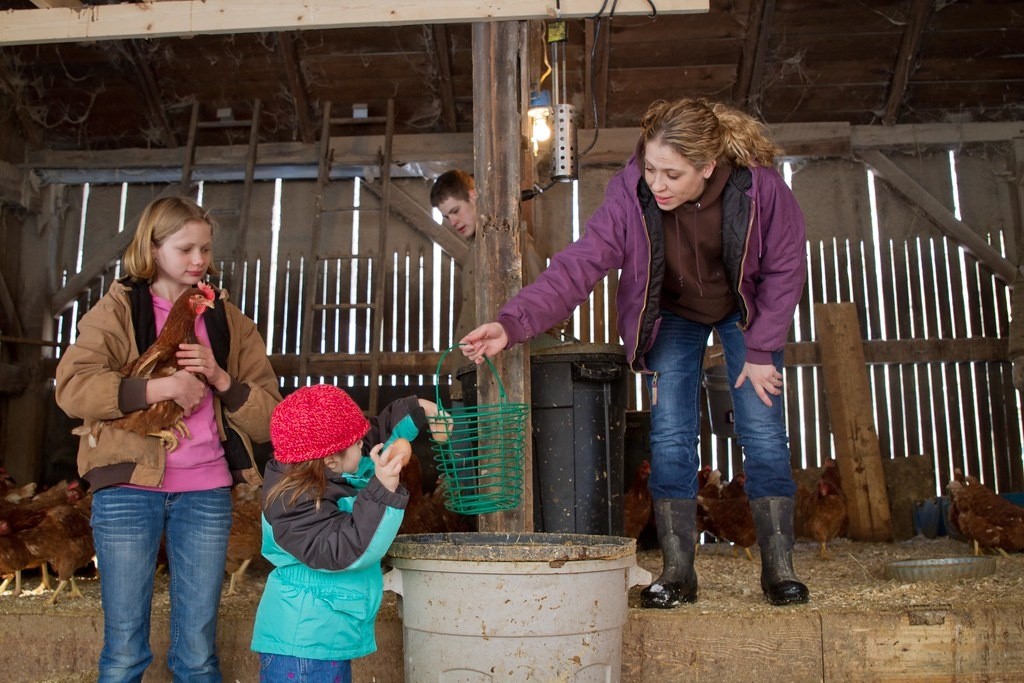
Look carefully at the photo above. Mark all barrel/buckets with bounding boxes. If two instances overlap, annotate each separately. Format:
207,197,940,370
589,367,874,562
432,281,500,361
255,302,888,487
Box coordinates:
382,531,636,683
456,353,629,537
702,365,737,438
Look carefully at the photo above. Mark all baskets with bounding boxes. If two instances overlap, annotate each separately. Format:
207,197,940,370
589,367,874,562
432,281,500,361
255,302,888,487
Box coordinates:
425,342,530,515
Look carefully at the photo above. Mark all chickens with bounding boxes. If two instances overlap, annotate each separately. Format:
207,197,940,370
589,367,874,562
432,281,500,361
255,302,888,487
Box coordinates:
68,280,216,457
0,451,1024,608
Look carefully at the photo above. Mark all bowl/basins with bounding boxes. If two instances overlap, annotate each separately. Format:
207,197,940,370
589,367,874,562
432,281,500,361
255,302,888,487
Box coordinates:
884,556,994,581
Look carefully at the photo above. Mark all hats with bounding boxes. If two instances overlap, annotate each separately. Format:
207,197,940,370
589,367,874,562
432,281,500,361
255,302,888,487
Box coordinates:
271,385,372,465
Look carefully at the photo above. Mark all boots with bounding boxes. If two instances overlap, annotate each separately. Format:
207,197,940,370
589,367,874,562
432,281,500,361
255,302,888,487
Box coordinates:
640,499,697,608
750,497,809,605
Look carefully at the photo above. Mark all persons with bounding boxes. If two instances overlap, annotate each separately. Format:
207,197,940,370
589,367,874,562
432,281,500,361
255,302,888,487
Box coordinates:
250,384,454,683
56,196,284,683
459,96,809,608
430,171,561,499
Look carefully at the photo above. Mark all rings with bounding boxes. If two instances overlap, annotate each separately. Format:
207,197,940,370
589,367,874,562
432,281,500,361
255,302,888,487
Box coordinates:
771,378,776,383
